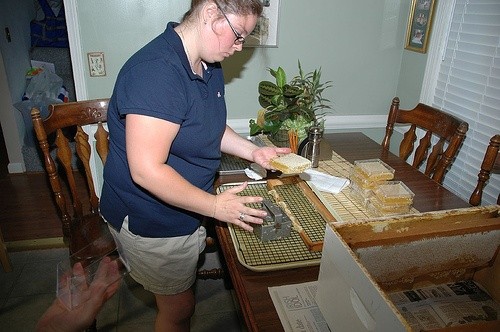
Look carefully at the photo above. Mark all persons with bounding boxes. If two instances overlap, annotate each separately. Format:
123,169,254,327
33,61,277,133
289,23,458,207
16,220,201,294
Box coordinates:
99,1,292,331
35,257,120,332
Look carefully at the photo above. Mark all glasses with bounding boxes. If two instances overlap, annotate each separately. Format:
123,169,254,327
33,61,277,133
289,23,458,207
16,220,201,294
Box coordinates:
214,0,245,45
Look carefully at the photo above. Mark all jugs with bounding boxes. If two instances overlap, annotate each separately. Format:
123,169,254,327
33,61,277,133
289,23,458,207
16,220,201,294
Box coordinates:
297,126,325,168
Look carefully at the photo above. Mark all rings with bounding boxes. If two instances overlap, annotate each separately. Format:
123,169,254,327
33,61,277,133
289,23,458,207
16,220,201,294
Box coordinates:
240,212,244,221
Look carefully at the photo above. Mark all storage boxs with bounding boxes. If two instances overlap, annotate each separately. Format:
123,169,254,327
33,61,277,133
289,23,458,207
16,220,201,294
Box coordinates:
314,205,499,332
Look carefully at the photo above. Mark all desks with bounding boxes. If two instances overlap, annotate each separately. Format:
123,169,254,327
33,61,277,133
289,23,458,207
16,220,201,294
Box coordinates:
214,132,472,332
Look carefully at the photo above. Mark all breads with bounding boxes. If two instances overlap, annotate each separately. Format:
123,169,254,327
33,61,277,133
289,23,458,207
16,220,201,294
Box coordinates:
269,152,313,174
350,162,413,215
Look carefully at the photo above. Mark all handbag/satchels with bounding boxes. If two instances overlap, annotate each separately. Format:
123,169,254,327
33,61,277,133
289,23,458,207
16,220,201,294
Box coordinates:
24,65,63,99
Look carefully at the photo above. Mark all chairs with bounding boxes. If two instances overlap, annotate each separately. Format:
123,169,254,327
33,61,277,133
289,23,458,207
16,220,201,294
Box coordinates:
31,98,117,332
468,134,500,206
381,97,469,184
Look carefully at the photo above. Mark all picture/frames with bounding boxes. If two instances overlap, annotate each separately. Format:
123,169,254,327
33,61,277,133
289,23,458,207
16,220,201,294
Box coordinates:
404,0,438,53
242,0,282,48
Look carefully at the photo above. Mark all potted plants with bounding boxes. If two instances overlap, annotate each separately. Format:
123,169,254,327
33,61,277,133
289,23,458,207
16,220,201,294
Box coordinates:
249,59,332,148
